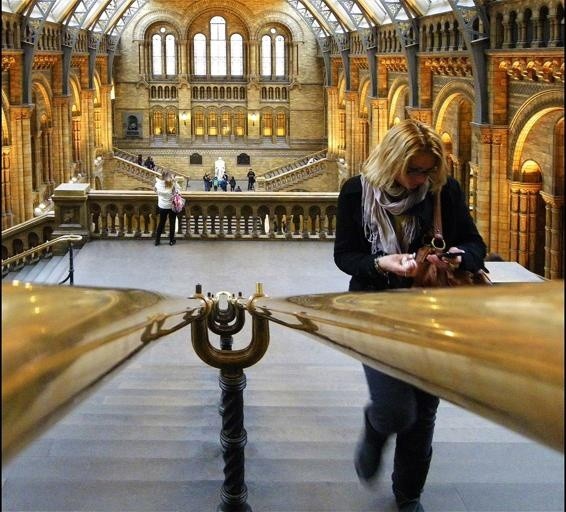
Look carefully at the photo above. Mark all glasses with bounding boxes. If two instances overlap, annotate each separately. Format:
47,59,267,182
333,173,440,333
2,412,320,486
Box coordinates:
405,163,437,177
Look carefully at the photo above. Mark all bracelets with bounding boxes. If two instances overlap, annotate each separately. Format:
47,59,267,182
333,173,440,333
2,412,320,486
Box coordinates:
373,254,389,275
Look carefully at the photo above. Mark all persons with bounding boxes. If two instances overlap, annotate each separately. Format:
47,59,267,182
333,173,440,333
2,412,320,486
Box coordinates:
153,169,182,247
332,117,489,512
307,157,318,166
202,167,256,193
337,157,345,190
95,156,104,189
214,157,226,178
137,154,155,171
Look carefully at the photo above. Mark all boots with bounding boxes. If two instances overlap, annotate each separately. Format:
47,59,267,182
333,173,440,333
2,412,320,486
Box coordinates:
391,446,434,511
355,416,392,480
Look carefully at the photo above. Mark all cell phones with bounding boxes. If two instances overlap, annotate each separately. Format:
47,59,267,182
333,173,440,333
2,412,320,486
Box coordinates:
437,252,464,259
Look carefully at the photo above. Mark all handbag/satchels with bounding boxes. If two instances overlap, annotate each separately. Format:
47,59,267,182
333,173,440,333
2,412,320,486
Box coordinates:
416,250,493,285
170,194,186,212
